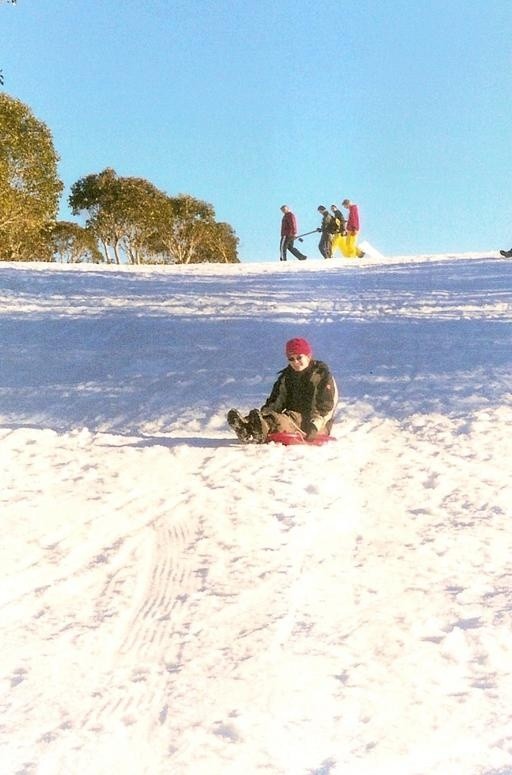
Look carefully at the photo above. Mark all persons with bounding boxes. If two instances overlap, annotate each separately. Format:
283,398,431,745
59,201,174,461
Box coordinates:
316,204,334,259
331,205,350,258
500,247,512,258
226,337,338,444
342,199,365,258
279,205,307,261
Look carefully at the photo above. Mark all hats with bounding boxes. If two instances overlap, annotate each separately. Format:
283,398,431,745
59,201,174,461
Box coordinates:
285,338,311,358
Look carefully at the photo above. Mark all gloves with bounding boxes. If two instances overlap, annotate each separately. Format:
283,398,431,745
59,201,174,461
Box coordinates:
304,421,318,439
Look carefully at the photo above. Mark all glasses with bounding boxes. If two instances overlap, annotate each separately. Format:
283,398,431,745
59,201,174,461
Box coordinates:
288,356,303,361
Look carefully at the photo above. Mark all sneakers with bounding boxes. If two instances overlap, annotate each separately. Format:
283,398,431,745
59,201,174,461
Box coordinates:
249,408,269,444
226,408,253,444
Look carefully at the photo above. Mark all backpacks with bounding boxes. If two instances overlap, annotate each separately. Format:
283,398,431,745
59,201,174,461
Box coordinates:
329,215,340,235
